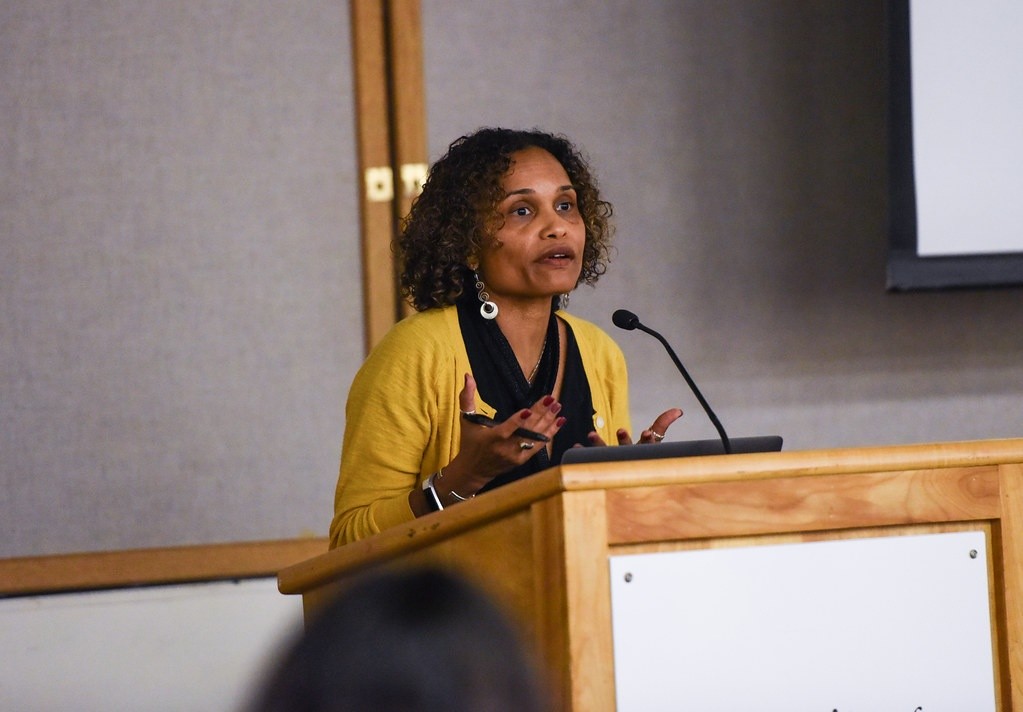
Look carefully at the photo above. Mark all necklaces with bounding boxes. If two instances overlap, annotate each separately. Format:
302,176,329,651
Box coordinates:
526,327,548,385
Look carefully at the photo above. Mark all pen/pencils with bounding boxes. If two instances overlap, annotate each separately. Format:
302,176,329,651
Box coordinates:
463,412,555,442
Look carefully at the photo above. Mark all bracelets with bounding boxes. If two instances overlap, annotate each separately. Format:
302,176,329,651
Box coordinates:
421,471,443,514
436,466,476,503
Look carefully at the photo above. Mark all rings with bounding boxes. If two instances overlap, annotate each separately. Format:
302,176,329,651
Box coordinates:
459,407,478,415
518,440,533,449
647,427,666,441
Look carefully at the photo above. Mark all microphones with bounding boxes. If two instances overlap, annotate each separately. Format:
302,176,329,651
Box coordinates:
612,308,733,455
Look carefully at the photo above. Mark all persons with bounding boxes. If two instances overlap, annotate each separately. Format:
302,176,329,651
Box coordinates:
327,128,683,554
247,564,550,712
